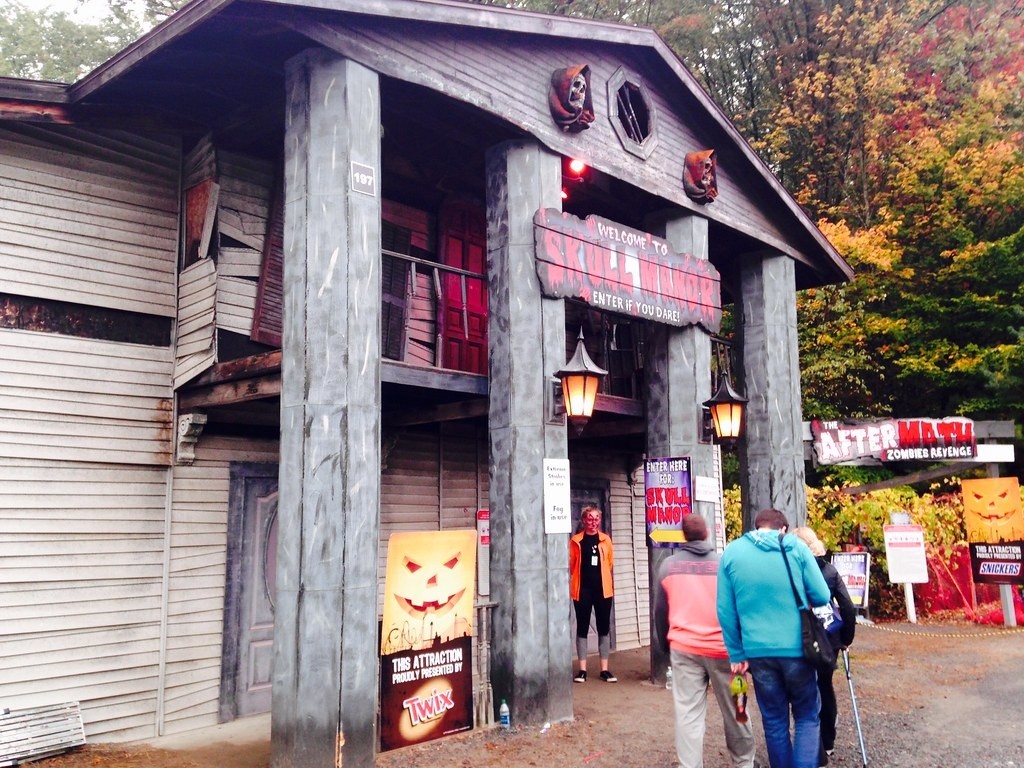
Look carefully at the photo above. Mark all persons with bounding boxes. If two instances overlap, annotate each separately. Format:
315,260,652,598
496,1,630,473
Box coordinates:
715,510,831,768
788,526,857,767
569,505,619,682
654,510,760,767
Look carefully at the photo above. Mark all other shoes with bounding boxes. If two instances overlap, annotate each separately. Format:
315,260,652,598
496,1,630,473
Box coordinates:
826,748,836,756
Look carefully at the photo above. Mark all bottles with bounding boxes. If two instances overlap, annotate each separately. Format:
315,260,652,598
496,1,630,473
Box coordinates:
734,692,747,722
500,699,510,730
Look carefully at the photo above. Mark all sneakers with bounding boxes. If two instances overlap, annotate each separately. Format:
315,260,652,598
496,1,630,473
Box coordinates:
574,670,587,682
599,670,617,682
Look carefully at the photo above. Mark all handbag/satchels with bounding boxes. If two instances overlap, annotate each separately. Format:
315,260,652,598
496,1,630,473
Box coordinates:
800,608,835,671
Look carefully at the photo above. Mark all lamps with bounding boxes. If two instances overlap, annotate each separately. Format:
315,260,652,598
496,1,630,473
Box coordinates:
702,369,750,443
544,326,609,427
